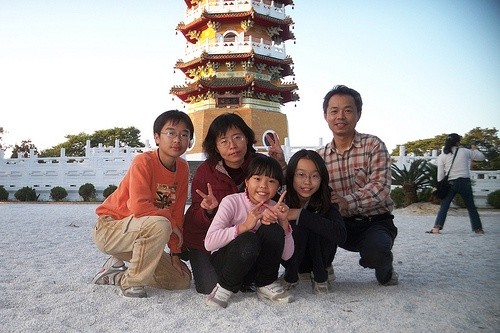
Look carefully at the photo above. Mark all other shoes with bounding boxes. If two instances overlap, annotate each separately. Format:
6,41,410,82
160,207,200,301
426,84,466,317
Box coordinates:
240,285,258,294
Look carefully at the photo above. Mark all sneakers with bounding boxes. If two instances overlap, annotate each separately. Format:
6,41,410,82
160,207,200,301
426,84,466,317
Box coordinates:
310,267,335,282
206,283,234,310
313,281,329,297
257,281,295,306
92,256,128,285
278,275,300,291
122,286,147,298
382,272,399,286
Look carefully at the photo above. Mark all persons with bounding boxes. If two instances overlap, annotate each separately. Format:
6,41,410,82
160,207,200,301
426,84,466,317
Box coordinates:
92,109,191,299
183,84,402,310
425,133,485,236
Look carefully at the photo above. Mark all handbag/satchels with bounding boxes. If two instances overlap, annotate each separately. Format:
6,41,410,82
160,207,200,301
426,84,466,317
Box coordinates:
436,176,451,199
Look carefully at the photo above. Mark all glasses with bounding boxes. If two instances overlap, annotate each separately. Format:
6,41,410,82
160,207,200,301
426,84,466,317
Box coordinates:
295,171,321,183
216,135,245,147
160,130,190,140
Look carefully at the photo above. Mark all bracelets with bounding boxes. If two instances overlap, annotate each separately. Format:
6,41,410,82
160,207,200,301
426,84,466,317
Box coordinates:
168,252,181,257
280,162,287,170
